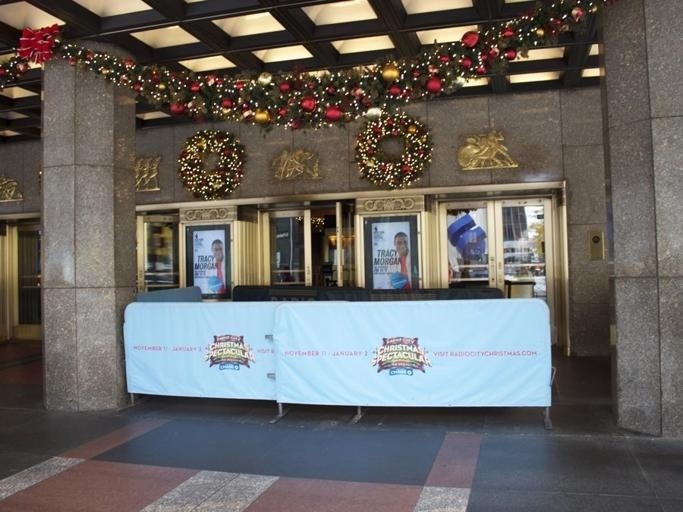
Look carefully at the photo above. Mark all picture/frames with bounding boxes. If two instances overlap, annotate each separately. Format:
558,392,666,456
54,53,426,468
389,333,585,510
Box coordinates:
363,216,418,289
184,224,231,298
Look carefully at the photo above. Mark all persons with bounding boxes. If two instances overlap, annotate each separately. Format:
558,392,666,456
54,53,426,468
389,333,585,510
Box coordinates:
205,240,226,293
374,227,384,246
195,234,204,252
386,231,412,290
